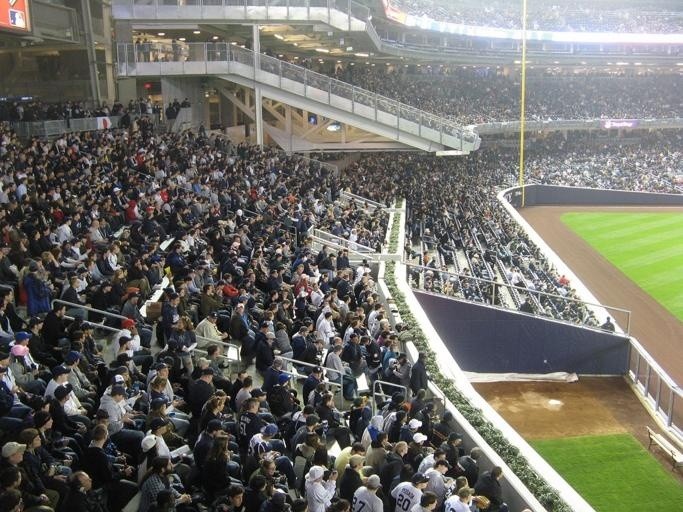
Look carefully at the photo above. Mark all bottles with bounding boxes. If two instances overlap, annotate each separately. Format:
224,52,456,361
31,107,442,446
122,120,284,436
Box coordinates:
123,463,135,478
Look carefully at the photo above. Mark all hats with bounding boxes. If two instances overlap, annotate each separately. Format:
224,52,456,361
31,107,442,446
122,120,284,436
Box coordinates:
1,114,483,512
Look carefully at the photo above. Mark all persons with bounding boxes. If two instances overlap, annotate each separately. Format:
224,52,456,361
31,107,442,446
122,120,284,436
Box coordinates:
0,0,683,512
